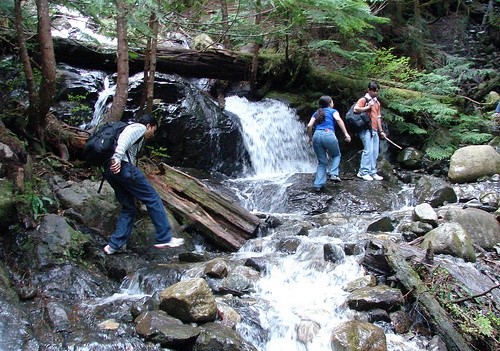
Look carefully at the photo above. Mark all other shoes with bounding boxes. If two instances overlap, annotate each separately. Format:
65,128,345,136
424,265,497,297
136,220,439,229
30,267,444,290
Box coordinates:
154,237,185,248
371,174,383,181
315,187,322,192
104,244,126,255
357,173,374,181
329,176,341,183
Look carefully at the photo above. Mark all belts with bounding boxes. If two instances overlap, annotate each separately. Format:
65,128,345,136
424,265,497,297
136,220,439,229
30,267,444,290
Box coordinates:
319,129,325,130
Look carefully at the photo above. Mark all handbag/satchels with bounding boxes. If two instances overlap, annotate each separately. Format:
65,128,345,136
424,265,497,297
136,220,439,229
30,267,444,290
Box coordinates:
345,97,372,132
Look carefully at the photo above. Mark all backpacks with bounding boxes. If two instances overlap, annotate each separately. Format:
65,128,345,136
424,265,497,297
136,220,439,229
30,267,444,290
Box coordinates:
82,120,143,161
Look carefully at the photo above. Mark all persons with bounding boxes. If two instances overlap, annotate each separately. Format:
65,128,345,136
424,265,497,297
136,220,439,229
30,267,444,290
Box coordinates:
354,81,386,181
102,113,186,255
307,96,352,192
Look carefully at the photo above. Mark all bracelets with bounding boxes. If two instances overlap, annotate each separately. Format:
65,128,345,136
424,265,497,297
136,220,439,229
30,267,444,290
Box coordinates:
378,131,384,135
